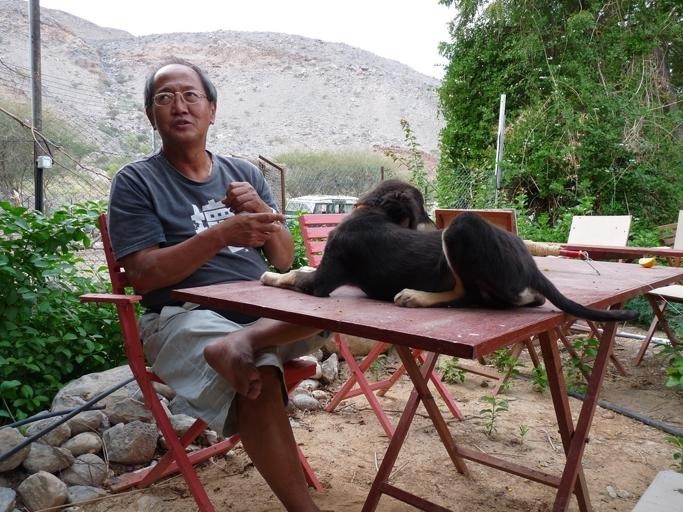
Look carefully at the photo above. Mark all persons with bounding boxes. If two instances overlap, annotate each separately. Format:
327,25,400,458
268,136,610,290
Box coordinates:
105,55,332,512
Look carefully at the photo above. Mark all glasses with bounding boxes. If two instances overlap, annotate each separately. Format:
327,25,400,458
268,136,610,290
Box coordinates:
146,90,211,106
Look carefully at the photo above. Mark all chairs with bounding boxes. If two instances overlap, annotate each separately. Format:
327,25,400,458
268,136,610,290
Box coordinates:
547,215,632,378
299,213,462,440
433,209,543,395
78,211,322,512
632,209,683,365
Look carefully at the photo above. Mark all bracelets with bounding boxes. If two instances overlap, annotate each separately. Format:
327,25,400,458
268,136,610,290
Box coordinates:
268,205,279,224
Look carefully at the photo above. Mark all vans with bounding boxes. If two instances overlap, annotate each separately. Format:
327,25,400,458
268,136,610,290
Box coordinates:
285,195,359,232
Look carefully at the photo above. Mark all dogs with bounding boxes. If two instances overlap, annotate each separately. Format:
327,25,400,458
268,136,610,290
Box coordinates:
259,177,642,322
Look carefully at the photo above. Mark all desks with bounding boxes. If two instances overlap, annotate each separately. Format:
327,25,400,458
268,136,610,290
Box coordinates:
539,242,683,377
167,257,683,512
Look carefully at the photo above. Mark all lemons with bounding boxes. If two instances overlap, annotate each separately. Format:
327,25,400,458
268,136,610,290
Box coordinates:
639,258,657,268
